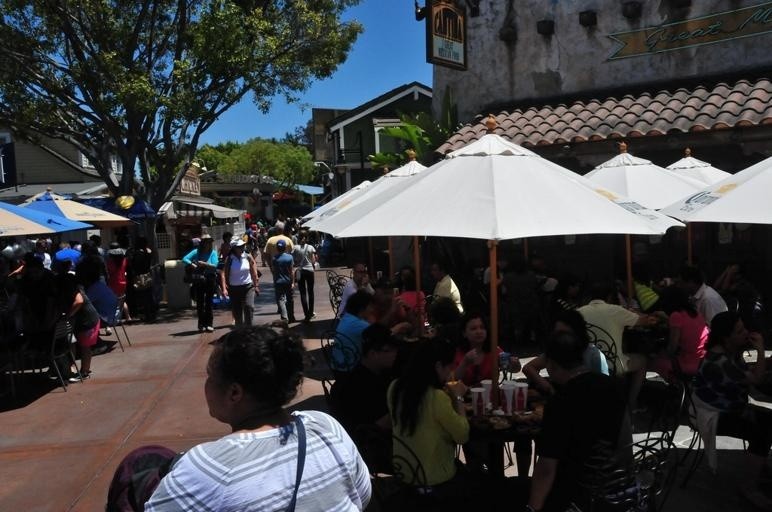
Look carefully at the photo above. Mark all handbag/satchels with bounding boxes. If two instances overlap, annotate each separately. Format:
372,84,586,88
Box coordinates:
293,244,308,282
184,261,206,282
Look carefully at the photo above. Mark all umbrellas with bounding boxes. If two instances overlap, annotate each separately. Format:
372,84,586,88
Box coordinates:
301,149,428,293
0,202,94,237
309,118,686,349
303,179,375,266
581,141,709,309
19,187,133,231
655,157,772,225
666,147,732,268
316,168,394,280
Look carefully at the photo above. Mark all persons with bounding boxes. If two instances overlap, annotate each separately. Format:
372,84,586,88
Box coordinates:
272,240,295,324
0,236,21,252
551,275,581,311
455,311,502,382
219,232,238,257
714,263,753,303
246,224,258,261
429,261,464,314
77,241,109,286
145,324,371,512
330,332,400,439
24,258,63,340
124,237,154,325
695,311,772,499
575,284,649,417
85,274,118,353
338,264,375,321
291,234,316,321
524,309,609,512
656,285,711,379
258,228,268,268
183,234,219,333
105,242,132,324
221,236,260,328
531,252,558,292
387,338,471,491
34,239,52,271
482,255,509,297
680,265,729,327
331,291,374,375
59,275,100,382
265,226,294,274
248,217,300,232
54,241,82,265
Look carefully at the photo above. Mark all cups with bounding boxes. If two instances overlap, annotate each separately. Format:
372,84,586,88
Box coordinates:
481,379,494,390
446,382,457,393
499,383,516,418
513,383,529,417
471,388,489,421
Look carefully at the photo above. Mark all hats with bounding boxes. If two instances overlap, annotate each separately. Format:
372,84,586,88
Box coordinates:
277,240,285,248
201,235,213,242
230,239,245,246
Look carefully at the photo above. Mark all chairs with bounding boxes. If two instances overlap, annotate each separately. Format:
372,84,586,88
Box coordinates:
100,294,131,352
585,323,618,374
325,269,353,315
353,423,427,512
43,315,84,392
677,380,746,491
321,329,361,397
590,437,678,512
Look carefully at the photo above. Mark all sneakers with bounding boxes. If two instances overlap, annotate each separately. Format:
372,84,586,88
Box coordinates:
78,370,93,374
311,314,317,317
199,326,206,332
304,318,310,321
207,326,214,331
76,375,89,379
